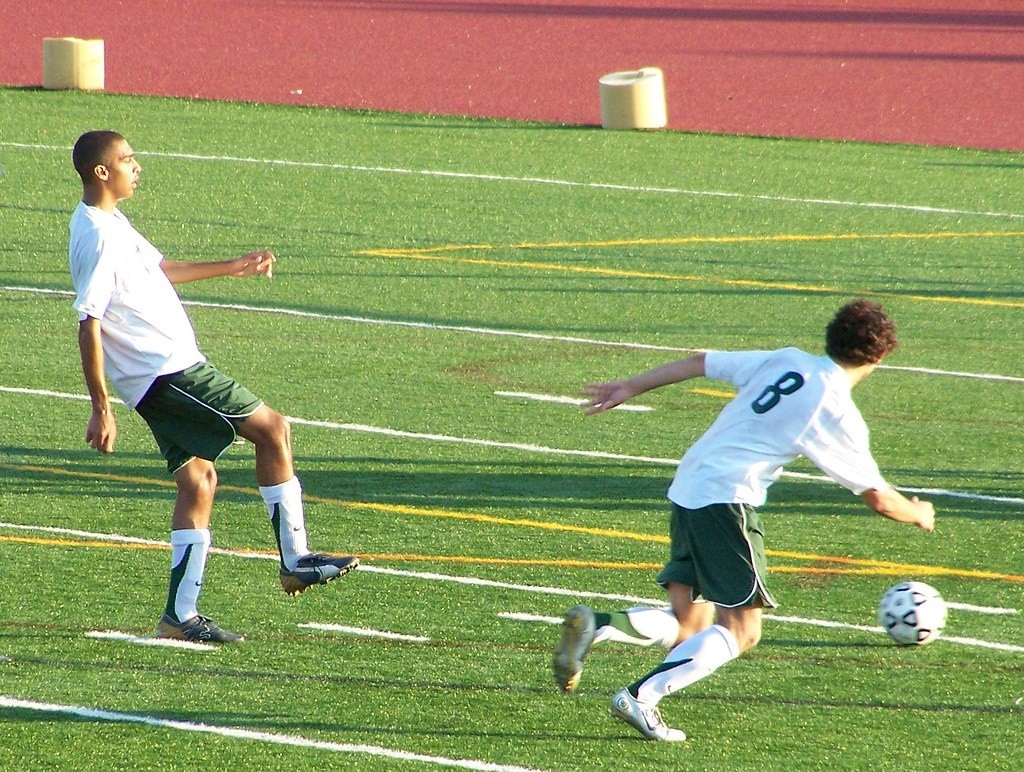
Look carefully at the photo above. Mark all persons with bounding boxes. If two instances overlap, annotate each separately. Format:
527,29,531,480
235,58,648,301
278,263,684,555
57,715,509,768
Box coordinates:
68,131,361,644
552,301,935,740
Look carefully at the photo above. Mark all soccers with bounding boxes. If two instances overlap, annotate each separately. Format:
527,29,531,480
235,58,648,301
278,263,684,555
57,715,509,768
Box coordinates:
878,582,949,646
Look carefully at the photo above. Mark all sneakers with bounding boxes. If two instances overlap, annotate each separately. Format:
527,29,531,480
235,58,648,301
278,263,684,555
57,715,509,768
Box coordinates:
280,553,359,597
156,614,244,643
552,606,595,694
611,687,686,742
